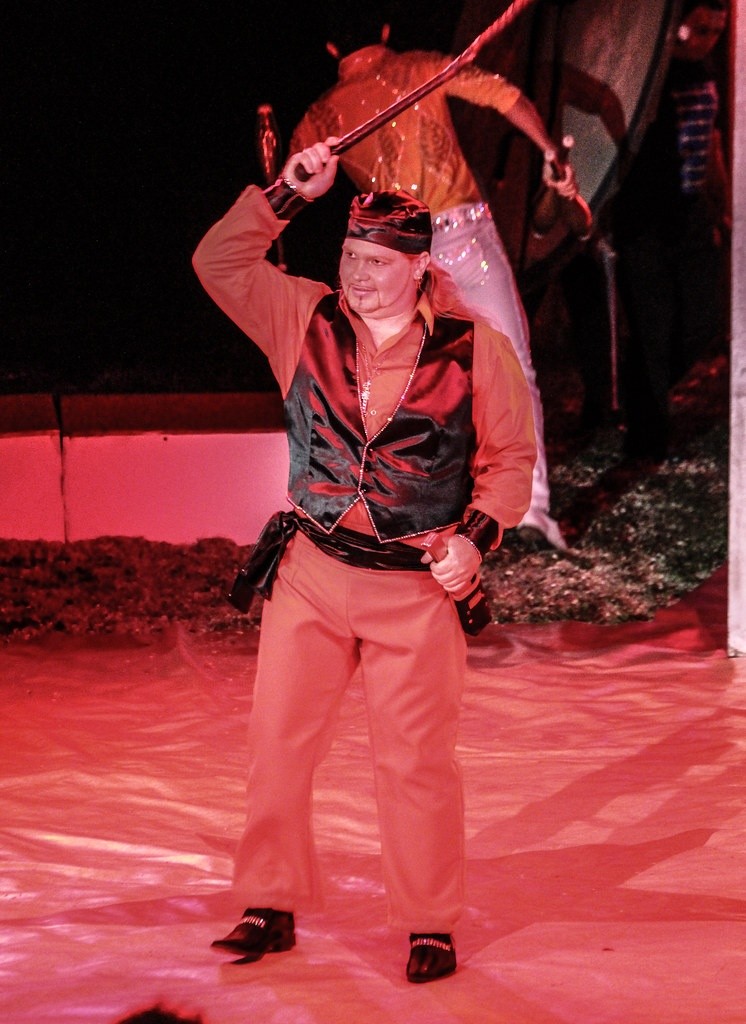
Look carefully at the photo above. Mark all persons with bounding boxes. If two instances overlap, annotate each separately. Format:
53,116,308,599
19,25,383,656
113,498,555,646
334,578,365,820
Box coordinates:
615,1,731,462
191,134,535,983
286,2,578,552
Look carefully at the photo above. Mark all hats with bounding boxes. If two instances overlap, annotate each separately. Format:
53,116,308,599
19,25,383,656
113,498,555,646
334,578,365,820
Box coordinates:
345,189,432,255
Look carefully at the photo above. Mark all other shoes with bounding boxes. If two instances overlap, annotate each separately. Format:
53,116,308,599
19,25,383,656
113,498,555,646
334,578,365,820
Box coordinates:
518,526,552,555
627,440,693,460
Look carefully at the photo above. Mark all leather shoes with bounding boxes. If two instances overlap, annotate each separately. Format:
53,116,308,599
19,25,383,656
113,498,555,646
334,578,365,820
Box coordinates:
404,937,456,982
212,907,297,954
